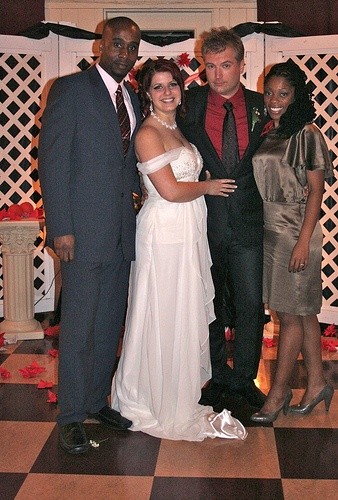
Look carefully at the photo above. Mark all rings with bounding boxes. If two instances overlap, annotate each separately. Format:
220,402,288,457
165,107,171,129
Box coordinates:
300,264,305,266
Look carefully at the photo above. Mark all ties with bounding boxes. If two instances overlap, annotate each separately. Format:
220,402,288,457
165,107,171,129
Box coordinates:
222,102,240,178
115,85,131,158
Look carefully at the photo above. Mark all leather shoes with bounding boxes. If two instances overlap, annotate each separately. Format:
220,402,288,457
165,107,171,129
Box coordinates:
88,407,133,430
58,422,89,454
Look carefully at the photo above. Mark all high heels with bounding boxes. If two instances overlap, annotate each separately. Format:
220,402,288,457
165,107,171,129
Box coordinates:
250,386,293,423
288,381,334,415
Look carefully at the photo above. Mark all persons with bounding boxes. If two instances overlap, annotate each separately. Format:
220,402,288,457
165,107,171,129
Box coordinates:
39,16,145,454
250,61,334,422
176,30,273,410
112,60,246,442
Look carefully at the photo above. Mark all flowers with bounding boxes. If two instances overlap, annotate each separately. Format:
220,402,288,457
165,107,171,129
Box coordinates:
175,52,191,68
250,107,261,131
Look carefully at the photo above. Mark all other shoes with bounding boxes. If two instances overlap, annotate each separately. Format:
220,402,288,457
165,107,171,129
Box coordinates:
233,383,267,409
198,382,230,408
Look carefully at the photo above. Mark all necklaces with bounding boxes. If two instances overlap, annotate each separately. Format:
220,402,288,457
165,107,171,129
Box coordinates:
150,111,180,131
